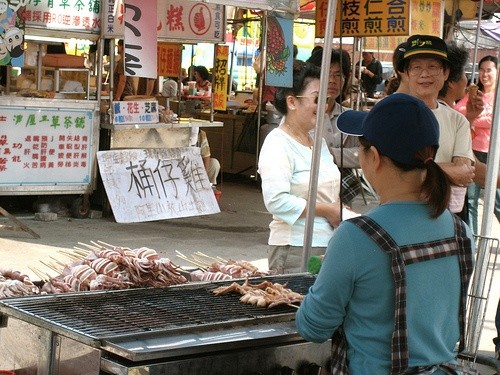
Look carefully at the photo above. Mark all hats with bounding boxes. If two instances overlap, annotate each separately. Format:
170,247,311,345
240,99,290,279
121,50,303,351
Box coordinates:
404,35,451,62
335,93,440,164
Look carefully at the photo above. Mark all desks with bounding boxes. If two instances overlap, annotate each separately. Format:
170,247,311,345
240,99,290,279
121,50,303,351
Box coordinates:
99,117,224,215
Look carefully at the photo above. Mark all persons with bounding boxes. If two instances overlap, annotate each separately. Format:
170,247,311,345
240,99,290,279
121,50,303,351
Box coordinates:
293,93,476,375
0,34,500,251
259,58,341,276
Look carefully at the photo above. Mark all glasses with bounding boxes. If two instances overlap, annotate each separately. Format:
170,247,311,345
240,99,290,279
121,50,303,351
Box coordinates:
296,96,332,104
409,64,443,76
